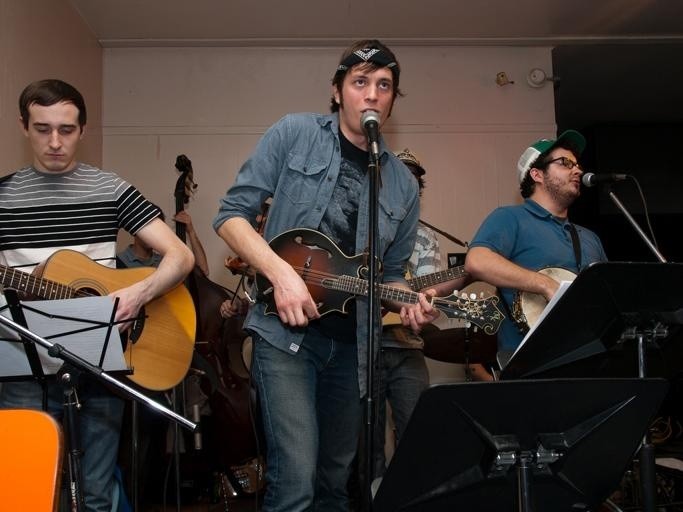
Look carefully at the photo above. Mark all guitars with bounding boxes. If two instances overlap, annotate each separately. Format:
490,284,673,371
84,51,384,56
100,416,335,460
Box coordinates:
252,228,505,336
0,249,198,392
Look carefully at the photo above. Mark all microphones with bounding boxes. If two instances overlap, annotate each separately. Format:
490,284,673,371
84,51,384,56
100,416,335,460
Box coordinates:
360,110,381,162
582,172,628,186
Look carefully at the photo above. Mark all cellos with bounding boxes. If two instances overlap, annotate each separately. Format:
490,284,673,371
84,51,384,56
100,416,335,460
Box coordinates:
172,154,264,471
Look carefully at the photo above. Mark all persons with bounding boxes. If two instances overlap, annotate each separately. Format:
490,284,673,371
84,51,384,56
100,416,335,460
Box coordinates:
464,137,608,383
214,39,441,512
469,331,500,382
217,202,273,322
0,78,196,512
373,150,484,492
113,204,210,512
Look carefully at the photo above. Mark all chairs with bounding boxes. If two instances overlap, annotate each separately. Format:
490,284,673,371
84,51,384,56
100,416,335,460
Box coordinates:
0,409,65,512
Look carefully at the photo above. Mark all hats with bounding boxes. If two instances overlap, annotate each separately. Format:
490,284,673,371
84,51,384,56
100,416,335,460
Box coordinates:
517,130,586,185
393,148,420,167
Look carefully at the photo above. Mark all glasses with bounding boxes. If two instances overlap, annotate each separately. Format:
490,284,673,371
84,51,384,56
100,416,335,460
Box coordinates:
548,156,582,169
